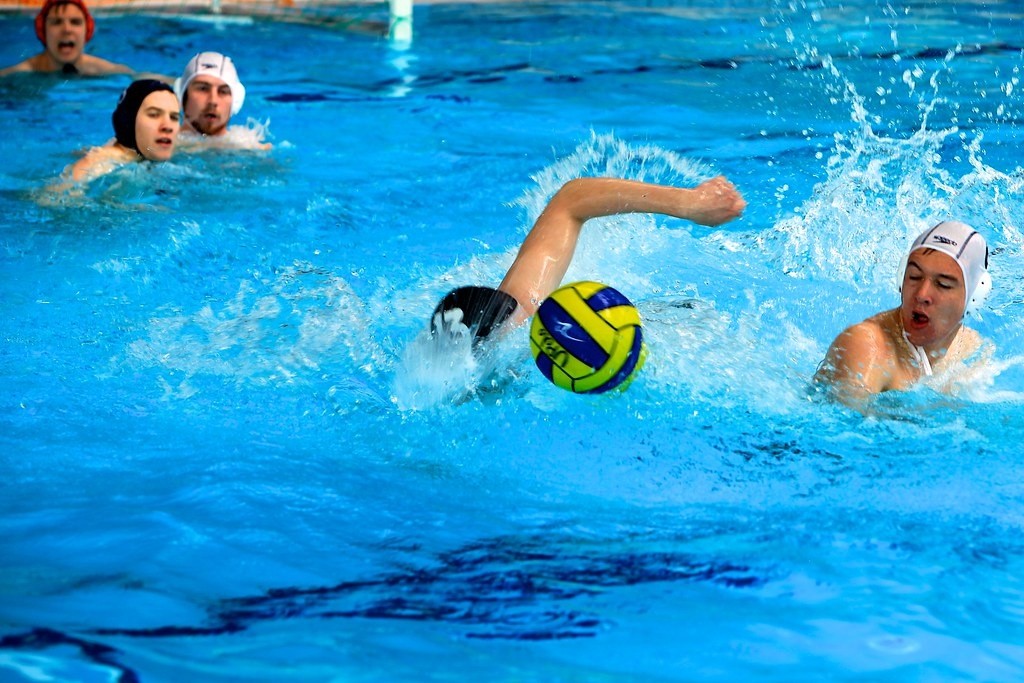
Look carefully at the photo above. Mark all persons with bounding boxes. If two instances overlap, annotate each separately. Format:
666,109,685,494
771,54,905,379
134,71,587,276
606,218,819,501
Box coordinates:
817,221,990,394
176,50,247,137
429,176,746,348
73,80,181,174
18,0,117,70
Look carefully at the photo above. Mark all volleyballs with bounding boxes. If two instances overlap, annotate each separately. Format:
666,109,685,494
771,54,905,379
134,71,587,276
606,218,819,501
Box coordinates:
529,281,643,395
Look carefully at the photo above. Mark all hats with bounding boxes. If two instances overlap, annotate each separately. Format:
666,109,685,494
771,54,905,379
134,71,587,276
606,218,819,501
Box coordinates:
897,220,992,319
430,284,517,349
111,79,175,155
33,0,95,47
173,51,246,117
